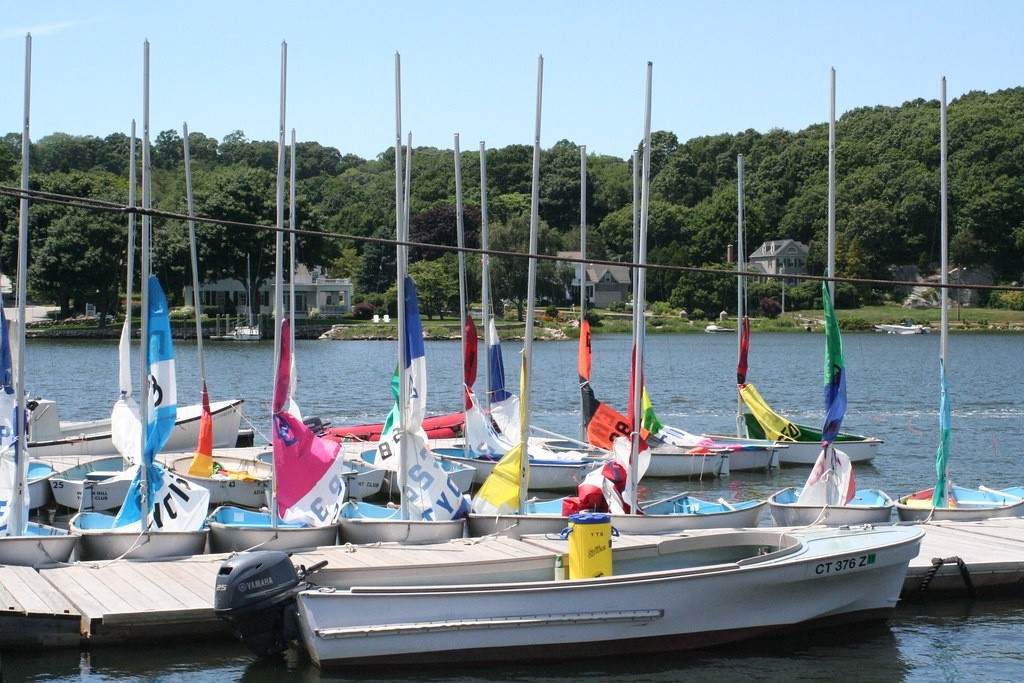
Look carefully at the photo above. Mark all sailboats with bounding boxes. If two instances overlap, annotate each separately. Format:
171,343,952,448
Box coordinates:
0,30,84,569
894,78,1024,521
334,51,468,544
205,41,340,555
224,253,263,341
22,119,885,516
67,39,210,559
463,54,584,536
606,62,768,536
764,69,896,530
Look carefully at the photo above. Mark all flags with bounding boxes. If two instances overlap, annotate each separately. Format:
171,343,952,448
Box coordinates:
796,266,857,507
1,288,29,538
577,316,879,452
374,274,474,521
110,272,210,531
457,314,587,461
273,316,346,527
929,355,955,509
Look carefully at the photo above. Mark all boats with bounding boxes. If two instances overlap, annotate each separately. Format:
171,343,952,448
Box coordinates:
704,326,735,334
876,317,930,335
5,394,258,459
210,524,925,669
